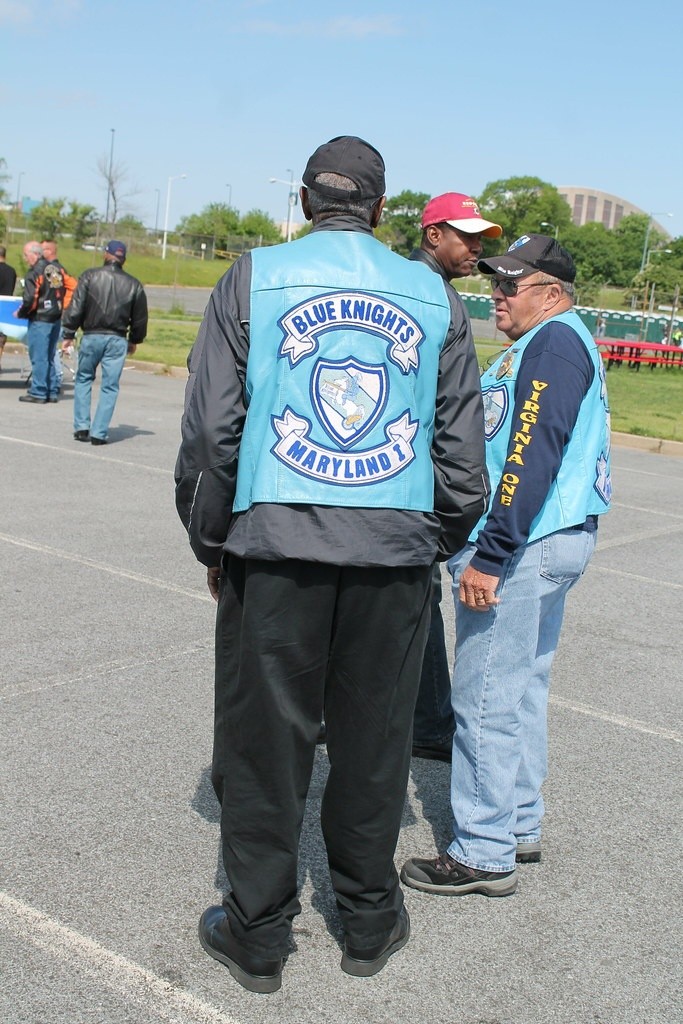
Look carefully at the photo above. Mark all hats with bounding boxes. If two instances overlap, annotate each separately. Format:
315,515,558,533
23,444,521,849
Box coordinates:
477,234,576,284
422,193,502,238
105,240,126,261
302,136,386,201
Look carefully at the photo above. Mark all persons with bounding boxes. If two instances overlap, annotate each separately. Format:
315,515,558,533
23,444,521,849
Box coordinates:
596,316,607,337
176,136,491,994
0,245,16,354
401,234,612,897
661,324,683,349
61,240,148,445
315,194,500,766
13,241,71,403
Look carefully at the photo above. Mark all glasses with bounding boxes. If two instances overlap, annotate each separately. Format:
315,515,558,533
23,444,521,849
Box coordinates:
490,276,560,297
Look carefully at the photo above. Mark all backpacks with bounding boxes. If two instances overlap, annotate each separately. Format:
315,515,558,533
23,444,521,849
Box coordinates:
61,267,78,310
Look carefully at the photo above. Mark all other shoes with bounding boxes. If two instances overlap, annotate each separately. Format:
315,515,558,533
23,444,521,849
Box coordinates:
411,731,454,764
315,724,327,744
19,394,46,404
46,398,58,403
91,437,106,445
74,430,89,441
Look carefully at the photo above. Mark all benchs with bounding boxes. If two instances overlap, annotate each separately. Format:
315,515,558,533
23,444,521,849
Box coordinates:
600,350,683,364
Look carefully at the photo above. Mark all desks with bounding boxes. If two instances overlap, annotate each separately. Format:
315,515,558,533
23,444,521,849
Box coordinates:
595,339,683,372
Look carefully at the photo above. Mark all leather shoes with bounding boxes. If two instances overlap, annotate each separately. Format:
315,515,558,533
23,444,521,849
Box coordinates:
341,904,410,977
199,904,283,993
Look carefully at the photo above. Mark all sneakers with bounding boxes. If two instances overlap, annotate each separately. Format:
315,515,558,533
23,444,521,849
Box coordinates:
516,840,541,863
400,850,518,898
